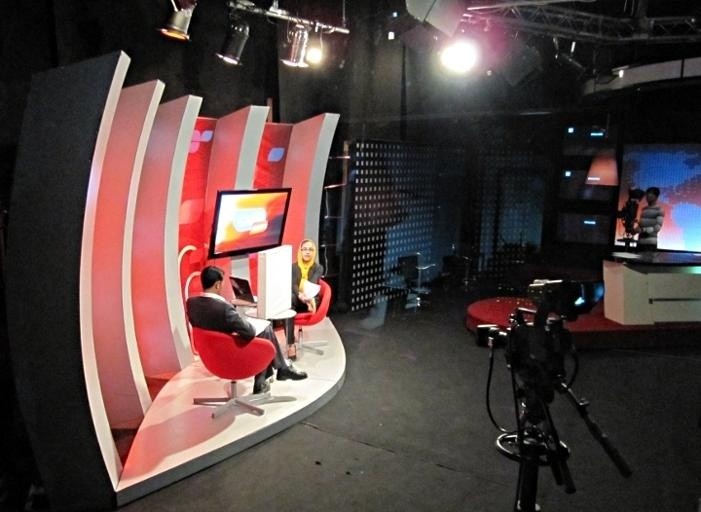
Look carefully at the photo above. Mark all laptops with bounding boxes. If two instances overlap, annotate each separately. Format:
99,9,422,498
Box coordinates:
229,276,257,303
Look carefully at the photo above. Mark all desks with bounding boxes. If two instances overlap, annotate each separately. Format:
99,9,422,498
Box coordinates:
245,309,296,368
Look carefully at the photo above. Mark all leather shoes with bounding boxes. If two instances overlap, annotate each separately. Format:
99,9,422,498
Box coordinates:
288,344,296,360
254,381,270,393
277,368,307,380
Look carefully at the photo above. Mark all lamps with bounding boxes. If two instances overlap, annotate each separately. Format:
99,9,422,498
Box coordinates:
158,0,197,41
217,0,350,68
549,36,586,80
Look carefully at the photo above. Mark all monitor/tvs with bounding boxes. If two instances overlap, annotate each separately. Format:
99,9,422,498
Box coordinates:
207,188,292,259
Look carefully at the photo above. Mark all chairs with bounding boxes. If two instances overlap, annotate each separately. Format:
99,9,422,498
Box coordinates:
191,327,277,419
294,279,332,360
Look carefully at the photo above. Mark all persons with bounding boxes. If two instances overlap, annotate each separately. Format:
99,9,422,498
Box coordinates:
631,186,665,251
185,264,308,394
283,239,323,362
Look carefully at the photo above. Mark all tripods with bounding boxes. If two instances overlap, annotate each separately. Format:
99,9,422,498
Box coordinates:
495,383,634,512
